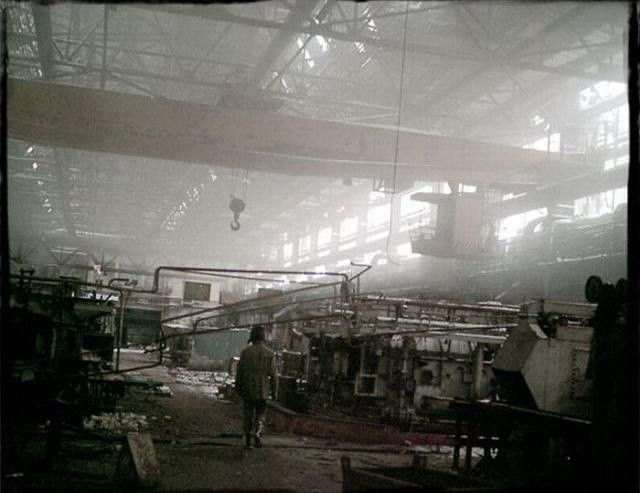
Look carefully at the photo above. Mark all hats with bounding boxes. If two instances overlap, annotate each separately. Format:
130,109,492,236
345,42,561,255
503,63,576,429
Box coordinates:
247,328,267,344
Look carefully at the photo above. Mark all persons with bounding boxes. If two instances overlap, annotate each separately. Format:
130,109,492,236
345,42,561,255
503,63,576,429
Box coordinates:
234,326,279,449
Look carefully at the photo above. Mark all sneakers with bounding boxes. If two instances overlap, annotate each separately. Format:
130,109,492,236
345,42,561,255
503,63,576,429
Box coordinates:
244,432,263,449
